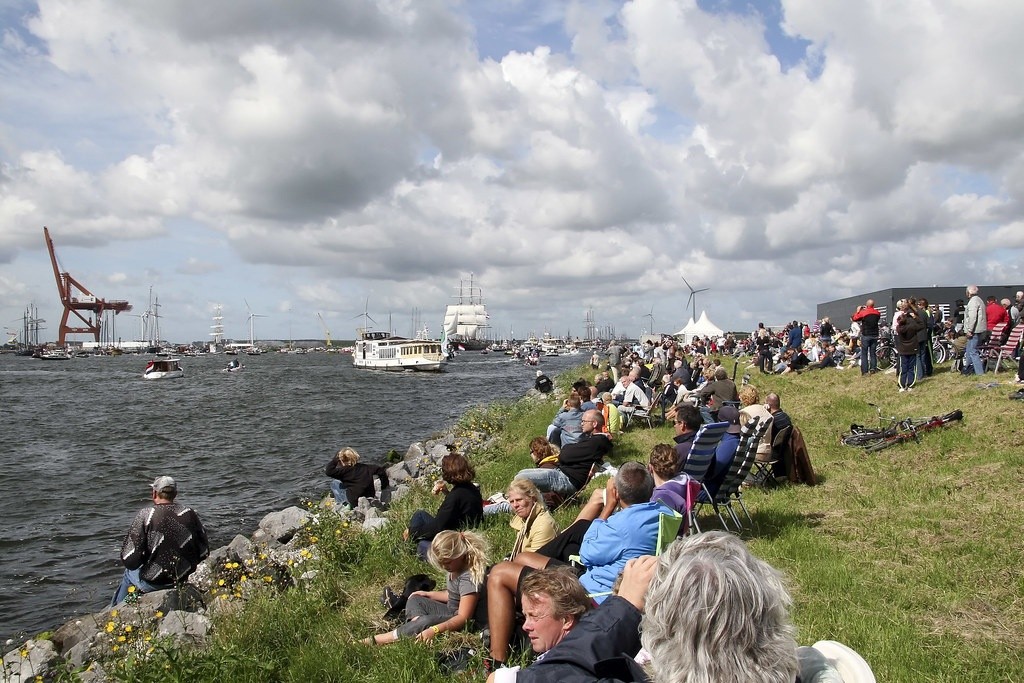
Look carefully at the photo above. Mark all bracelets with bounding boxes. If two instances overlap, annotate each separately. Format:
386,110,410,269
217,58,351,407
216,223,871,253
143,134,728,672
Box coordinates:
429,626,440,638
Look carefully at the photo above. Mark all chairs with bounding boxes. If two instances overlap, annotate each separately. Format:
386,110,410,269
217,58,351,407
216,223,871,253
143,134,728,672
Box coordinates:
552,321,1023,540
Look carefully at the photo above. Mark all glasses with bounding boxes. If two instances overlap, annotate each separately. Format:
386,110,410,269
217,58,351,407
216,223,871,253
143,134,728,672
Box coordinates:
673,420,684,425
582,419,592,424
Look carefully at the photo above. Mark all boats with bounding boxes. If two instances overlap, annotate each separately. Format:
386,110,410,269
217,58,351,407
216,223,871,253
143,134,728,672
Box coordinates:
144,352,183,379
13,341,208,360
223,344,355,355
222,357,245,373
481,309,616,366
351,331,449,371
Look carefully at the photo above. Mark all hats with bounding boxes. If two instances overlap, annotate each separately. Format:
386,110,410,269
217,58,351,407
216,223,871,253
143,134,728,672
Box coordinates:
537,370,543,377
149,476,177,496
718,405,741,434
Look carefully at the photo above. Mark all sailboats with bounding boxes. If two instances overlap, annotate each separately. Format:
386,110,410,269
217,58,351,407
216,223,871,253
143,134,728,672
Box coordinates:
442,272,492,351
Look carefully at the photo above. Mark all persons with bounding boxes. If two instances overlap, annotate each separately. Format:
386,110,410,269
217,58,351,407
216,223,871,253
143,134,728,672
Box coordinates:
110,475,211,608
326,446,392,521
354,285,1024,682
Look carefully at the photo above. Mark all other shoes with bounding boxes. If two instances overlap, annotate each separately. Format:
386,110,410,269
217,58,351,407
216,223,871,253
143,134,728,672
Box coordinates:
381,586,400,611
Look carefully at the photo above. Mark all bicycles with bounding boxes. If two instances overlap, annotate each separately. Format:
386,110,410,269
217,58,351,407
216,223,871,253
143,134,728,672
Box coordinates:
873,329,959,371
840,400,965,452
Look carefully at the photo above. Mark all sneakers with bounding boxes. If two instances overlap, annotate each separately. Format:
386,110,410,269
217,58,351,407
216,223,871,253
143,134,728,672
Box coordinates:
484,656,502,677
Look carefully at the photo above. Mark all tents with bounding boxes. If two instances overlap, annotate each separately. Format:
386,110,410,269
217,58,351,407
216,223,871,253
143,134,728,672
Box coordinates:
672,317,695,346
686,311,724,347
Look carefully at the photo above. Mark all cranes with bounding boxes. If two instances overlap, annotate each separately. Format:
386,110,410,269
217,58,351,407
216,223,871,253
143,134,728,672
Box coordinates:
42,225,134,350
318,312,333,346
